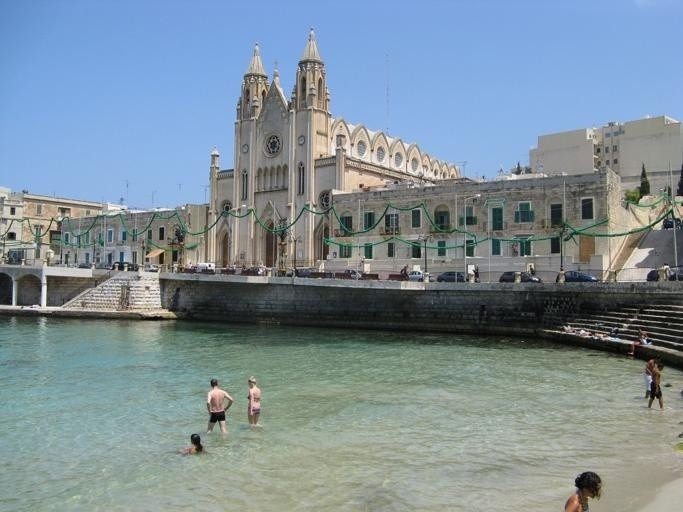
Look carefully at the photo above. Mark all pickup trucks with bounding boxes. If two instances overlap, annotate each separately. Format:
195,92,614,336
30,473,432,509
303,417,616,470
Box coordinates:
112,262,138,271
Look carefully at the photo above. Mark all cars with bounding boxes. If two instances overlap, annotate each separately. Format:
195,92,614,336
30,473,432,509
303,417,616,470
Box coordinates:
646,269,682,281
407,271,434,282
437,272,477,282
555,271,598,282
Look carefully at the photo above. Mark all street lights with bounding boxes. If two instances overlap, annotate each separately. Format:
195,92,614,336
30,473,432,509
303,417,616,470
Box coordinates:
58,217,63,265
558,226,564,271
422,236,429,273
232,204,246,261
463,194,480,282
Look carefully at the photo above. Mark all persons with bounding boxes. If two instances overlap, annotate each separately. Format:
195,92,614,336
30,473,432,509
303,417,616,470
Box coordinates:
206,379,234,433
529,264,537,276
247,376,261,425
187,261,246,274
473,265,481,282
565,471,602,512
400,264,410,281
645,355,658,400
648,363,664,409
177,433,203,454
637,327,652,345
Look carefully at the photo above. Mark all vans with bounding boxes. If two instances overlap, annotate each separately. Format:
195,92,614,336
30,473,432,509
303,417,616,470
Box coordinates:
343,269,362,279
189,263,215,273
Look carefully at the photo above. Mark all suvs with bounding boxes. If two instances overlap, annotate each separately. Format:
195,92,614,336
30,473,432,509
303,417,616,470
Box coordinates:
287,267,313,278
499,271,542,283
144,264,158,272
662,218,680,230
242,267,268,276
